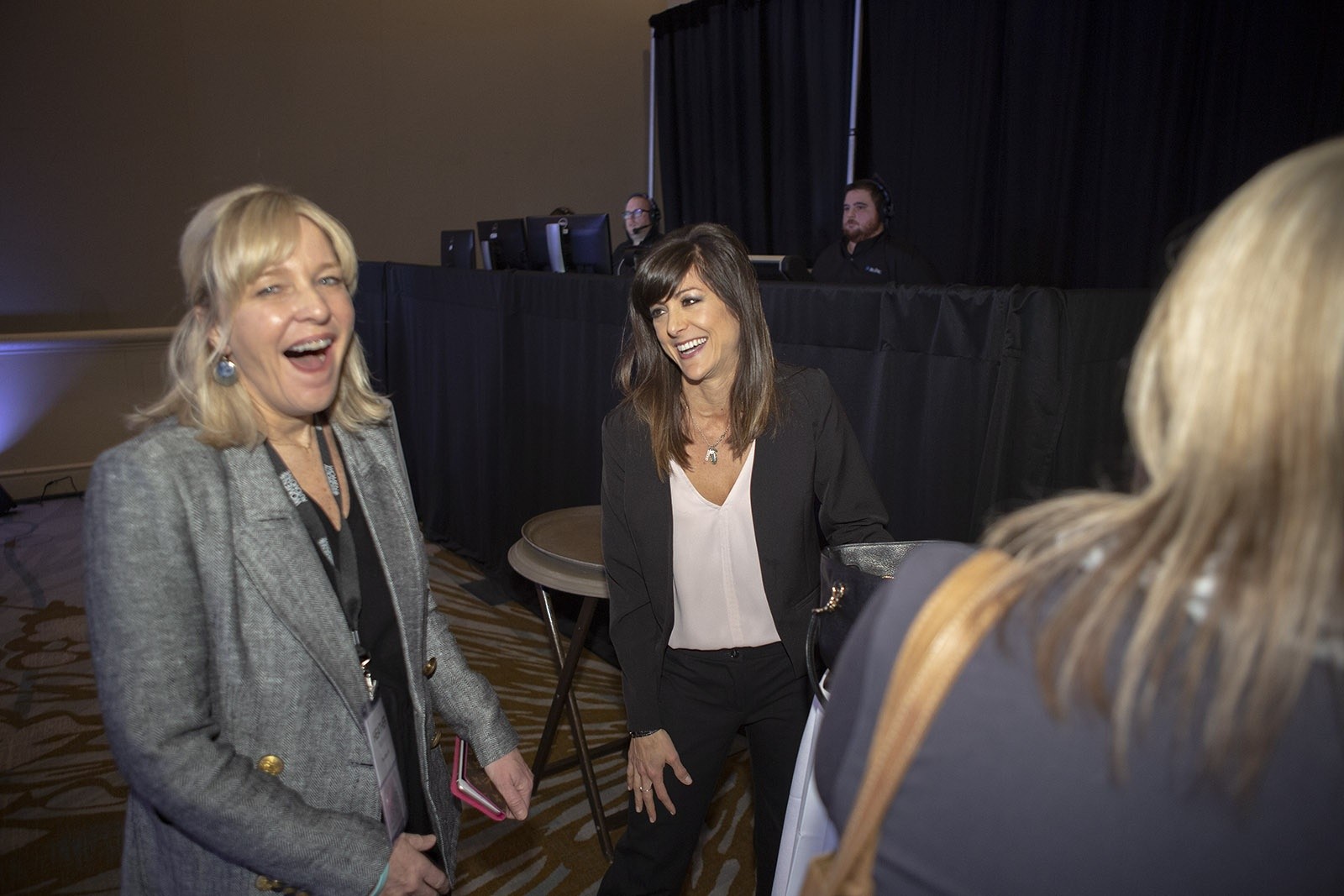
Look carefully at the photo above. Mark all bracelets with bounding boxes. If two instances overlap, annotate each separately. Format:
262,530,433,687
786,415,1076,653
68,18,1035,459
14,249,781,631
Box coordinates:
629,729,661,738
369,862,389,896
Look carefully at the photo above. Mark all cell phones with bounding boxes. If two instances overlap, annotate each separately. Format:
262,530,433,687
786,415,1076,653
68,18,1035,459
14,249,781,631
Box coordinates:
459,739,503,815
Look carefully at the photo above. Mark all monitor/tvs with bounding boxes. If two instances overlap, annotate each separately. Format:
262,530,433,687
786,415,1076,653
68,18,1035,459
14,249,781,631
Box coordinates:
441,213,613,273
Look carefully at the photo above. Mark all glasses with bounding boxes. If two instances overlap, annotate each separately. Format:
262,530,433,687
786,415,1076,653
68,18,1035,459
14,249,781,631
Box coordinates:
621,208,650,220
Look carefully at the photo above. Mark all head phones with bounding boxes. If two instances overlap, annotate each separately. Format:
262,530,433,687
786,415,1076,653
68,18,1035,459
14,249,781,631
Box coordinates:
862,178,892,216
627,193,661,223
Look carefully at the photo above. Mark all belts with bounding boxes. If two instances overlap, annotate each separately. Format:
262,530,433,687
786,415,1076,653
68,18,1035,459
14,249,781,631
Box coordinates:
671,641,781,665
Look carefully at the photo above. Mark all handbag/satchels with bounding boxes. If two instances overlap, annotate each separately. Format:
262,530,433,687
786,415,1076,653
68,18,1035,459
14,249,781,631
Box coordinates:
805,540,919,708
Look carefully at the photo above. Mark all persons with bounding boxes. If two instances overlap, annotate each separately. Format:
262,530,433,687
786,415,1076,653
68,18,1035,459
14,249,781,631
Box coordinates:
815,135,1344,896
82,184,534,896
811,175,913,284
595,222,896,896
612,195,668,278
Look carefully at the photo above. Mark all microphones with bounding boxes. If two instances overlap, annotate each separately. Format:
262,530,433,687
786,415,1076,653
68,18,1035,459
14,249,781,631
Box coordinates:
635,221,654,234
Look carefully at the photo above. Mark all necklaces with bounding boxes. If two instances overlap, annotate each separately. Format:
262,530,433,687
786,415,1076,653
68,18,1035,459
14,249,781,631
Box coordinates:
681,398,729,463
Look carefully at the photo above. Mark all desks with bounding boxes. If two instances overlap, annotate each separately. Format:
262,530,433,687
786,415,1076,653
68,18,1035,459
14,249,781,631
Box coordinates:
507,504,629,866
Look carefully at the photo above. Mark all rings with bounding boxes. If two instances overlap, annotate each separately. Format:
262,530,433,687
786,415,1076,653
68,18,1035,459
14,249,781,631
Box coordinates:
641,787,652,792
